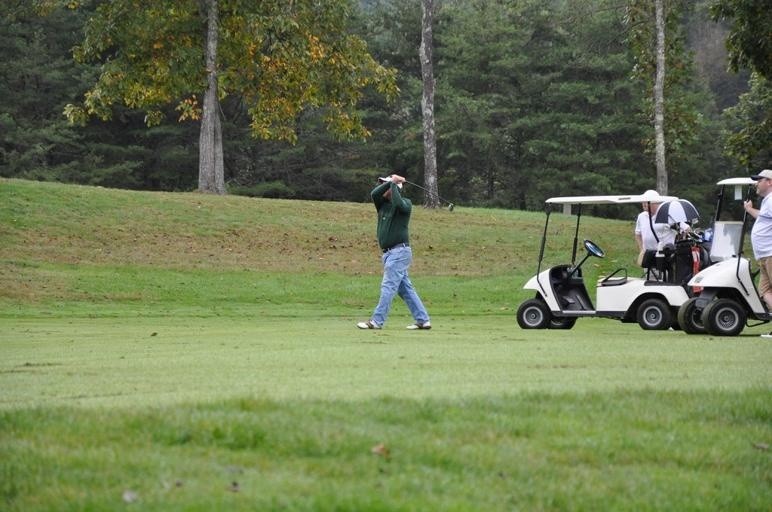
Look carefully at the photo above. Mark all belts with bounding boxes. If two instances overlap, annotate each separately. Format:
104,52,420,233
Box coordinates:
383,243,410,253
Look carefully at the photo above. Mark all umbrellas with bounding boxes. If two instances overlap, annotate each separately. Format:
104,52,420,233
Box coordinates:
654,197,701,242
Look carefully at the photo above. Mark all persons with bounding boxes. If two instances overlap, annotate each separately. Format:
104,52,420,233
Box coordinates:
356,171,433,331
744,168,772,339
634,189,691,284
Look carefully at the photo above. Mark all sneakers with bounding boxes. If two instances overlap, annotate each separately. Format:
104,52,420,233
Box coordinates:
357,320,383,330
406,321,432,330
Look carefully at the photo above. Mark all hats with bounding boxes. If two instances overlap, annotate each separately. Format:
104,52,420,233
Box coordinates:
751,168,772,181
378,173,403,190
642,190,659,195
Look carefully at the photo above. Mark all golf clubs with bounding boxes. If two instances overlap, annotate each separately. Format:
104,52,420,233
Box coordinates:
389,173,454,212
